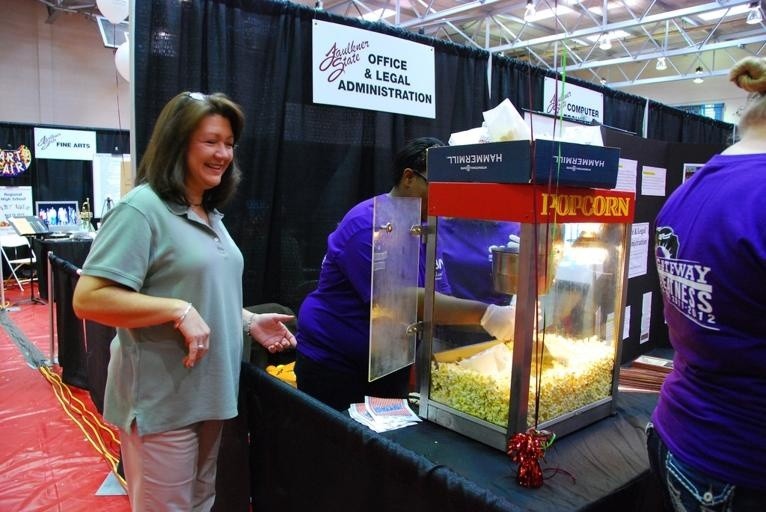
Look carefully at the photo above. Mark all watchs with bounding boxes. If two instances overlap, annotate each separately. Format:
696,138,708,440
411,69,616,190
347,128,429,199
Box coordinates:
244,312,257,335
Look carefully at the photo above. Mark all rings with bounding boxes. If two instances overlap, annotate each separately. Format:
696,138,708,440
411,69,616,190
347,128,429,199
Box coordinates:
196,340,206,353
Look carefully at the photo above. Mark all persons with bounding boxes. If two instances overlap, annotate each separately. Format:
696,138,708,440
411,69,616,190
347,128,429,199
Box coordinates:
413,214,544,352
294,138,517,413
72,88,297,510
644,56,766,512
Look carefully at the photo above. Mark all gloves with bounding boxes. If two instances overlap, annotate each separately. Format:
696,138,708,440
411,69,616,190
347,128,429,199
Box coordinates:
510,295,547,323
479,302,518,343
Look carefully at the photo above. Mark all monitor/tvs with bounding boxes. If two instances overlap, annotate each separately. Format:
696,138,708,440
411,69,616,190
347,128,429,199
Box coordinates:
96,17,129,49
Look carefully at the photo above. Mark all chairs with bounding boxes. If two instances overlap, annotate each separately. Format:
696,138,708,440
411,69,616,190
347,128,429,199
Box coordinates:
1,234,36,292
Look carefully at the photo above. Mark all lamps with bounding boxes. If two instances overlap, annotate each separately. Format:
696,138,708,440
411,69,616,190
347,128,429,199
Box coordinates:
656,57,667,71
599,32,612,50
693,67,704,83
600,77,607,86
524,0,536,23
745,3,763,25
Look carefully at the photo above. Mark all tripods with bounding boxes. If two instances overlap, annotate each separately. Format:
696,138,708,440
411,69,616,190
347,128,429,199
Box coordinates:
12,237,46,306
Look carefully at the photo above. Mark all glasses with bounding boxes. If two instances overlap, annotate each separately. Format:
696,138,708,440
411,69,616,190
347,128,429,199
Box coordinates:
413,169,427,183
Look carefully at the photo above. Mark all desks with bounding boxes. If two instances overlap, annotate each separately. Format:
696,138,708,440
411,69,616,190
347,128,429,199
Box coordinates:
217,349,674,511
33,236,93,302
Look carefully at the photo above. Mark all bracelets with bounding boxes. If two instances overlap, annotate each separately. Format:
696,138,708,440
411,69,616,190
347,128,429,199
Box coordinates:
174,304,196,329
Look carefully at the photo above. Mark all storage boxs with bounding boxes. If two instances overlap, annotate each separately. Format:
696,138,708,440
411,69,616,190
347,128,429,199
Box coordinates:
425,138,620,189
